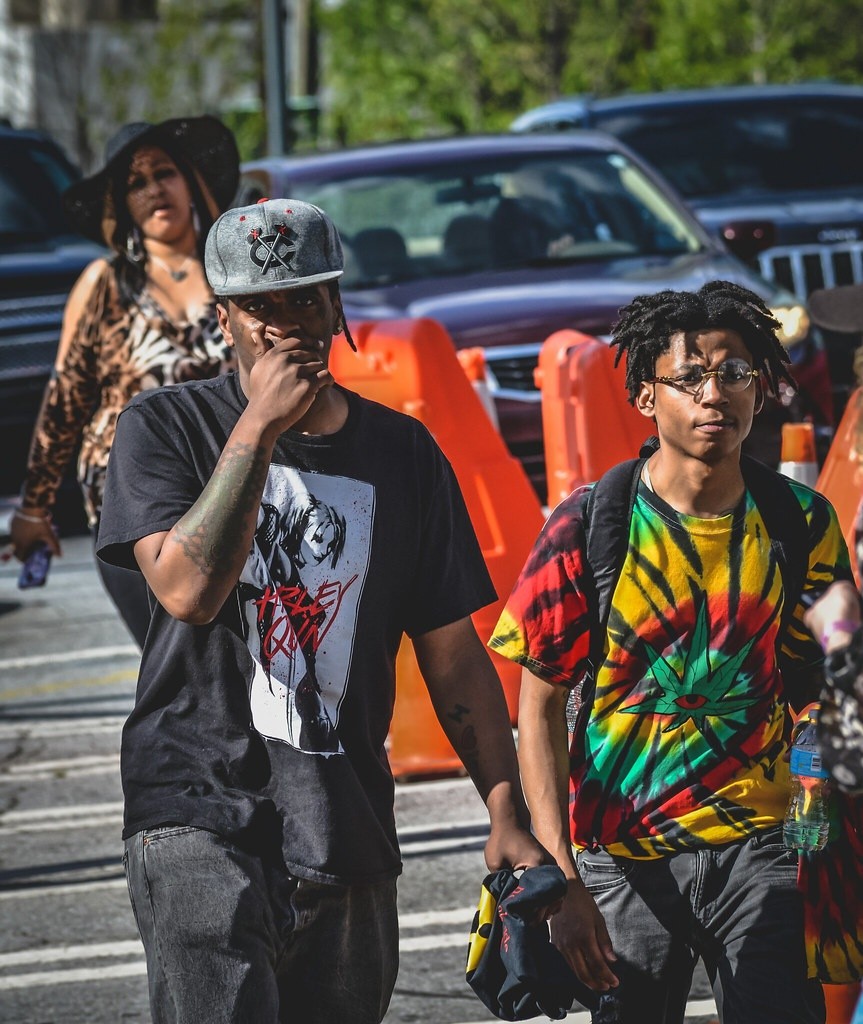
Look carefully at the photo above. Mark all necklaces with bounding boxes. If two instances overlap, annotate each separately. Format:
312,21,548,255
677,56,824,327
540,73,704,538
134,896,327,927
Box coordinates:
148,248,198,282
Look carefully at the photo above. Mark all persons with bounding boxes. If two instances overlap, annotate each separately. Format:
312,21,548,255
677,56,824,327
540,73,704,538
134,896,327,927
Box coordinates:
10,113,241,564
95,198,556,1024
487,282,863,1024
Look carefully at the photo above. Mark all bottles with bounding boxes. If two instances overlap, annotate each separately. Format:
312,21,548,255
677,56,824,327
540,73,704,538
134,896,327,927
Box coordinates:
782,705,830,852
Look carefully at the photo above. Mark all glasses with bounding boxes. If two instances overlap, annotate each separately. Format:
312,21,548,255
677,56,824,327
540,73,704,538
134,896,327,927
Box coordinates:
643,358,760,394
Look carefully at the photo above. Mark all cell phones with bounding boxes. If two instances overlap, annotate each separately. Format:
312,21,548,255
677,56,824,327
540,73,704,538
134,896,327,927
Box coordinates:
16,524,59,589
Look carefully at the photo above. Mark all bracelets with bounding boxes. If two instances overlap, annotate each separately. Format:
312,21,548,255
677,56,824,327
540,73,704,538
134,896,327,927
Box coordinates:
12,507,53,523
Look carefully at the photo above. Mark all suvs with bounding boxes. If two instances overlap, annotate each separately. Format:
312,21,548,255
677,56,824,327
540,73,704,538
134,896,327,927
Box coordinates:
227,126,837,497
508,86,862,344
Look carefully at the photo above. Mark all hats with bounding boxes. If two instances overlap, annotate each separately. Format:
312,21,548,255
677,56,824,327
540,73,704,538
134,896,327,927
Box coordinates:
204,198,344,297
66,114,242,248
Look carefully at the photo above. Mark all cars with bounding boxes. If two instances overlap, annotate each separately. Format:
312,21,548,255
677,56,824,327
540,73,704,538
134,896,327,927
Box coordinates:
0,114,117,501
